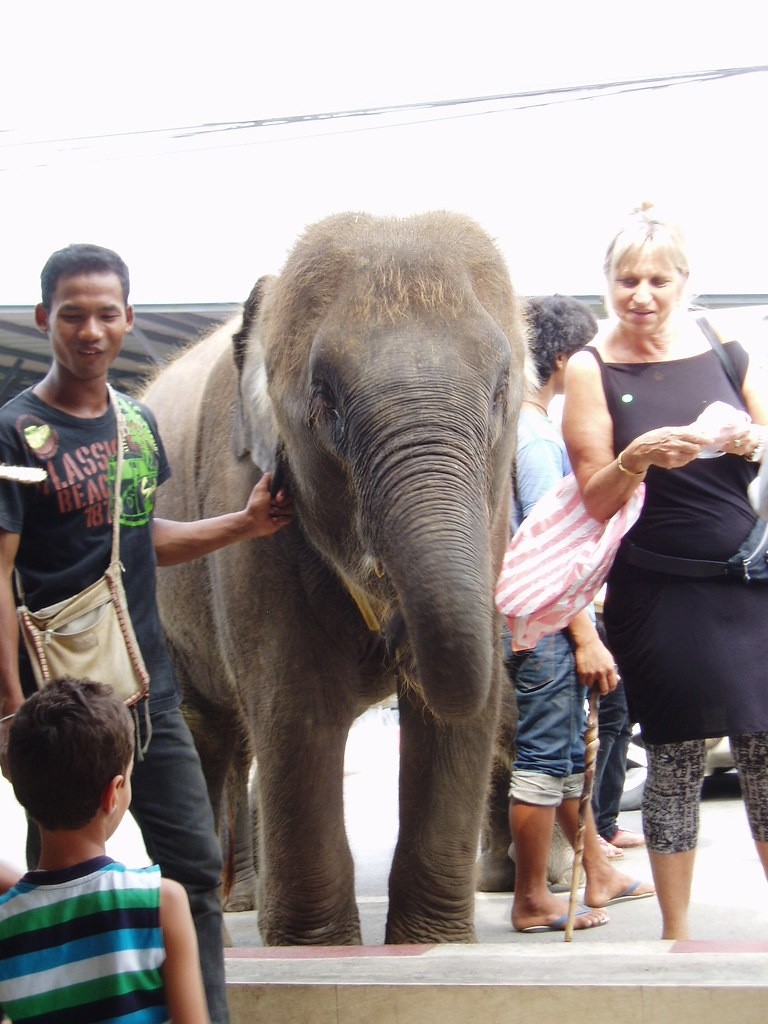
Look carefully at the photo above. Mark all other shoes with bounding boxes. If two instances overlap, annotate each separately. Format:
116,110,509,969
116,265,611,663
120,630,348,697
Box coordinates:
594,835,625,862
610,825,647,848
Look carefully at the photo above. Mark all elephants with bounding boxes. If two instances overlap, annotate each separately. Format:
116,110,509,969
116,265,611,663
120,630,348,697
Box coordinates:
135,212,538,947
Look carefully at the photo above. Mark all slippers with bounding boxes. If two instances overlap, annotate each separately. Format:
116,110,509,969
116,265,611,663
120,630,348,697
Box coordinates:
519,902,610,934
593,879,655,909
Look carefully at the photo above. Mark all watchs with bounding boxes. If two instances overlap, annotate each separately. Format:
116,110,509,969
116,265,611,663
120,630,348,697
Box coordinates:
744,426,765,462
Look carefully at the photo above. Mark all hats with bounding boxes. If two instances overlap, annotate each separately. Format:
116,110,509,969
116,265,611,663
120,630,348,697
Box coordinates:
494,469,647,653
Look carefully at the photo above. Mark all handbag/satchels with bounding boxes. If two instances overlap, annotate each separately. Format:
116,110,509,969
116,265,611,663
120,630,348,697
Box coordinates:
728,515,768,584
15,560,151,709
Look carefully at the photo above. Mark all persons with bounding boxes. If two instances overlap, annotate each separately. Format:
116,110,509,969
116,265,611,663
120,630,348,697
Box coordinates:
1,245,292,1024
561,216,768,936
502,294,653,934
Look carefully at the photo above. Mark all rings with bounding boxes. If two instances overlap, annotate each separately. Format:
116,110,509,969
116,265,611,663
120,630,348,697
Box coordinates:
734,440,740,448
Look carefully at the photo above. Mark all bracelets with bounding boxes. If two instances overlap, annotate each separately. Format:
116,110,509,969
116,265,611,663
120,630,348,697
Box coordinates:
618,451,647,477
